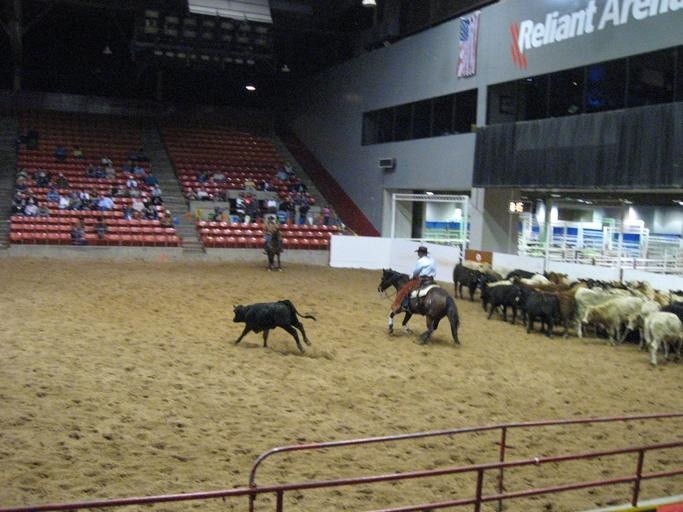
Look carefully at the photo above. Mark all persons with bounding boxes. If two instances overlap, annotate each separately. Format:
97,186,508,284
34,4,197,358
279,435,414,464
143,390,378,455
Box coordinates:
15,132,173,244
264,215,279,242
401,247,437,310
186,163,331,226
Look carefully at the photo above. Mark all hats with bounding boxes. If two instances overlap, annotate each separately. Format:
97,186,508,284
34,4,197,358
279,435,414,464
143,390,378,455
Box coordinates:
414,246,428,253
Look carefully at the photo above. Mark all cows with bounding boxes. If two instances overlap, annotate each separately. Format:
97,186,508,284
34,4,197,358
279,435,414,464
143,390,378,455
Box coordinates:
453,260,683,367
232,299,317,354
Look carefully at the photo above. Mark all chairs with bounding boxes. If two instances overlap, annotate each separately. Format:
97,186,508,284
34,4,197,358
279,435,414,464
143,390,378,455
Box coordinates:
8,116,346,250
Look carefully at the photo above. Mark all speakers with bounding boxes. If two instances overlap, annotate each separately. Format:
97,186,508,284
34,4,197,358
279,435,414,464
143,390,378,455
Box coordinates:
378,157,394,169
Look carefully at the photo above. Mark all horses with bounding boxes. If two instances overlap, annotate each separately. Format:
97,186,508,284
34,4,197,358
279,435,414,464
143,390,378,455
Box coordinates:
264,228,283,272
377,268,461,346
